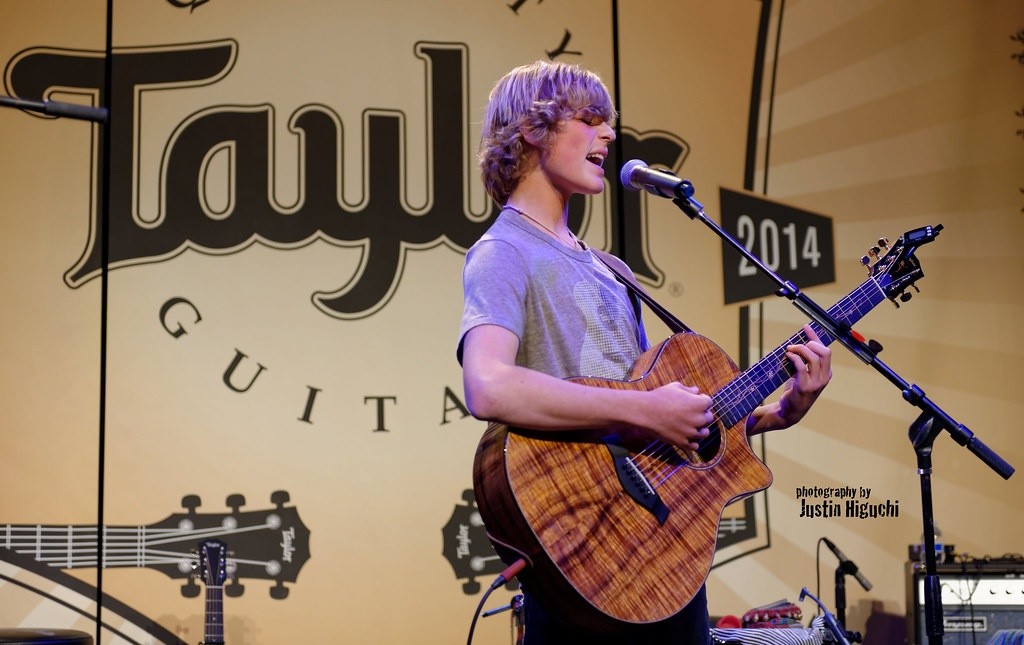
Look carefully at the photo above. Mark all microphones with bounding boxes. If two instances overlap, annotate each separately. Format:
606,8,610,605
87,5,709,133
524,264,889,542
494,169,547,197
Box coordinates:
823,538,872,591
621,158,695,198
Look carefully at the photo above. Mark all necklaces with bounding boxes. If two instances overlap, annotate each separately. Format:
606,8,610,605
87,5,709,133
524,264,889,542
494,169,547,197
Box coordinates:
502,205,579,250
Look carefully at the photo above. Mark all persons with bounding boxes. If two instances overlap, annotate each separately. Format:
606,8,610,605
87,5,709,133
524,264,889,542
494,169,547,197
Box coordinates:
455,63,833,645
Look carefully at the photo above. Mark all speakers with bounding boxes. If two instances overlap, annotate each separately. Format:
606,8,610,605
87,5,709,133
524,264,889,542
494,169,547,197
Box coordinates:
913,571,1024,644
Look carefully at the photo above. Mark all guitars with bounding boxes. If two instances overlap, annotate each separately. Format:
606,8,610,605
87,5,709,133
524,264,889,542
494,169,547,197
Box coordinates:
189,537,236,645
469,234,927,640
